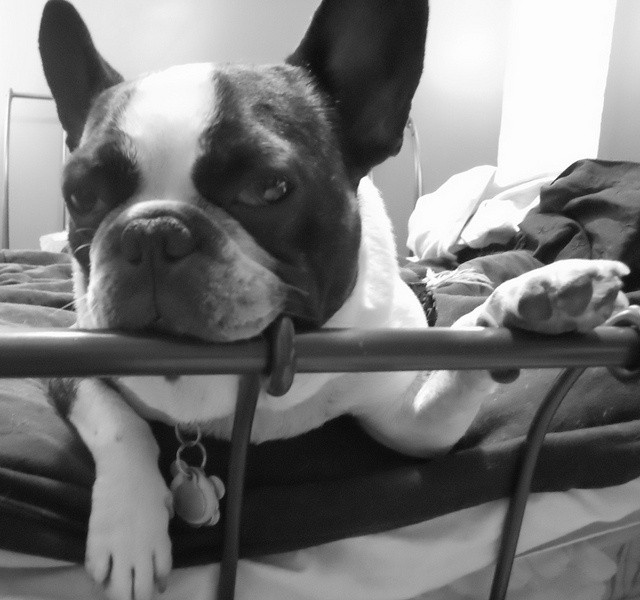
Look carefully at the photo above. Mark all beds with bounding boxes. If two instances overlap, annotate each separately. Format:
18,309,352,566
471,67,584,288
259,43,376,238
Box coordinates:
1,89,638,594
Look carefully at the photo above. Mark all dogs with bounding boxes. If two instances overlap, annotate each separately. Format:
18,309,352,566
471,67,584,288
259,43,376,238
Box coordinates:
39,0,630,600
